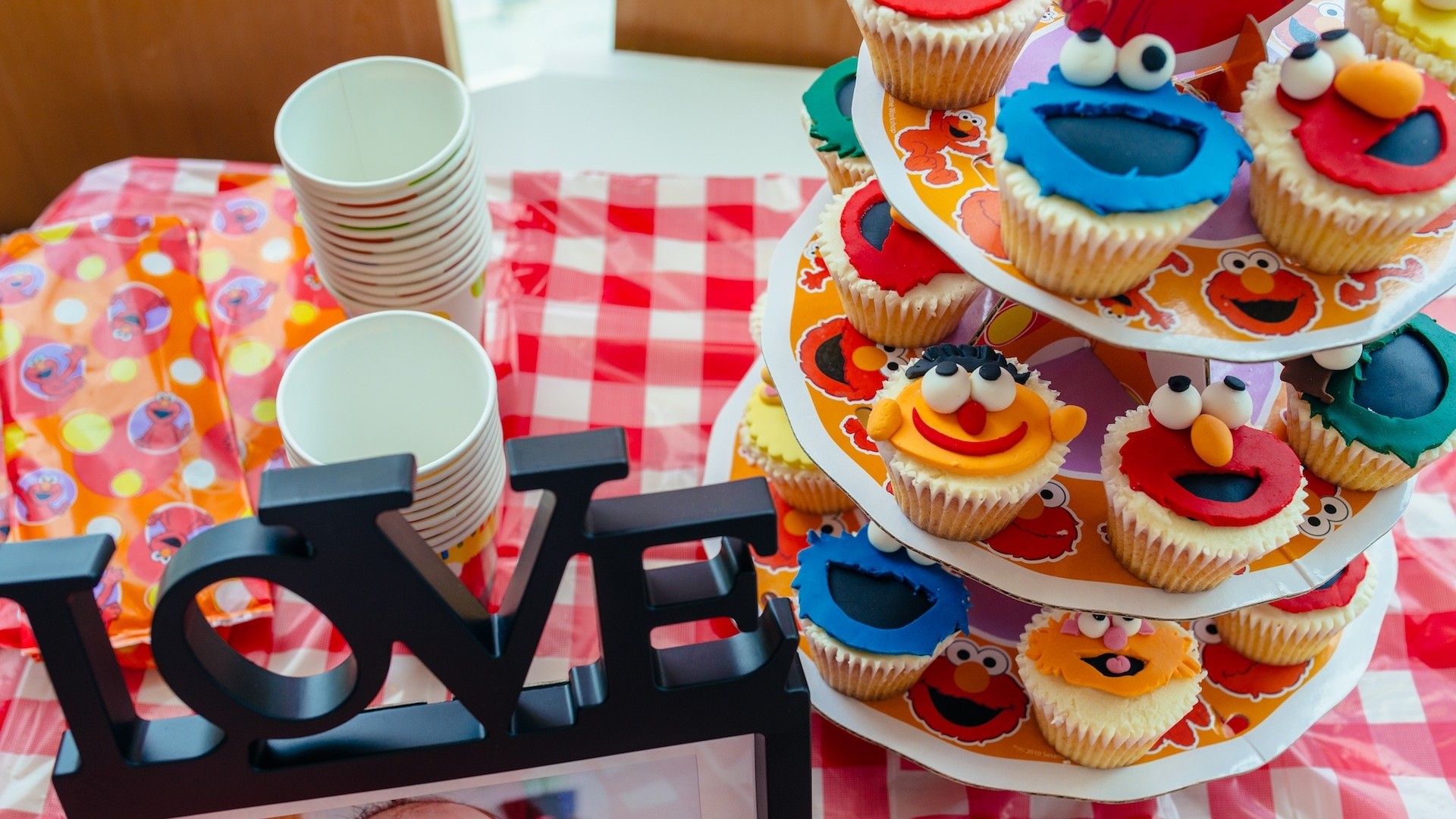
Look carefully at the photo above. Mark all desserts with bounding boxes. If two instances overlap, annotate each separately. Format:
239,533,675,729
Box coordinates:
736,362,1381,769
805,56,1455,595
847,0,1456,298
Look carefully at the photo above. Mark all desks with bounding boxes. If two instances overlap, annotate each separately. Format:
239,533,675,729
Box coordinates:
0,157,1456,818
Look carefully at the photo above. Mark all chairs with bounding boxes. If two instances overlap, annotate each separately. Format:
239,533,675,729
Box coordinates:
0,0,456,231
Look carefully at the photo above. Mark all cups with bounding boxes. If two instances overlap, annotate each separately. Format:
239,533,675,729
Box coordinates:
274,55,493,344
275,311,509,611
